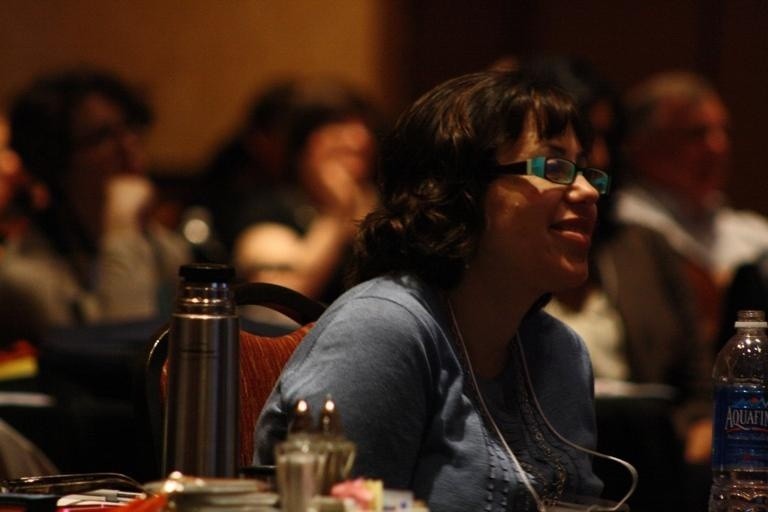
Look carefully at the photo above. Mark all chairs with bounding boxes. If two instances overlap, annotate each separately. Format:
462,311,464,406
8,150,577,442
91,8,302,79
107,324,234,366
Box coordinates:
139,281,328,482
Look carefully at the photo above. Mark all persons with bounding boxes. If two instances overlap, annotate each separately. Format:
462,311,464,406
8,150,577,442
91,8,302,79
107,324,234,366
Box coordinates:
2,70,388,357
253,55,645,510
539,71,767,387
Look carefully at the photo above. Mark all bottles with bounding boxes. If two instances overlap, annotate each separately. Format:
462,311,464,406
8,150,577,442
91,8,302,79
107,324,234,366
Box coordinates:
275,397,357,512
167,263,240,478
703,307,768,510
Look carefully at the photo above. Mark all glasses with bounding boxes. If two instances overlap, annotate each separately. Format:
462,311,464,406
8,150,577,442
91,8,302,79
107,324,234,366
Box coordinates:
490,156,612,196
